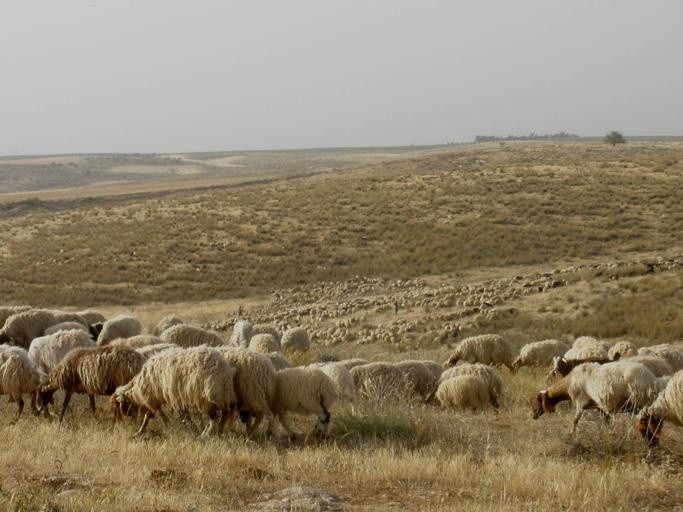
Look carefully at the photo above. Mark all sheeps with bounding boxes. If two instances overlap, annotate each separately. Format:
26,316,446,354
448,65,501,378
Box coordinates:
608,340,637,362
0,303,513,440
550,337,609,377
532,363,659,439
637,343,682,370
514,340,568,368
637,370,682,448
